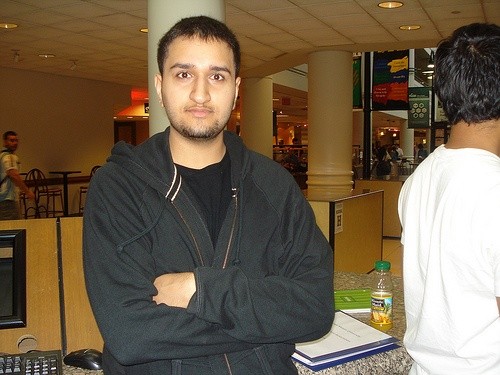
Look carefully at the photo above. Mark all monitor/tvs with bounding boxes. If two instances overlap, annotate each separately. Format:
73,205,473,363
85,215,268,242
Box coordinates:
0,228,26,329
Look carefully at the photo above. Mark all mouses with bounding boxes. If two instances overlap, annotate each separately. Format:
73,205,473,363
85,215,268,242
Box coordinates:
64,348,103,369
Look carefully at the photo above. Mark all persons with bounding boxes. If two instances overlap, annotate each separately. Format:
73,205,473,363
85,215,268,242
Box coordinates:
273,132,428,180
0,131,35,221
397,23,500,375
83,15,335,375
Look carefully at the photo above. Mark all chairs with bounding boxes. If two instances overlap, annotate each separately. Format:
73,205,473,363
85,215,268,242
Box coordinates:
19,166,103,220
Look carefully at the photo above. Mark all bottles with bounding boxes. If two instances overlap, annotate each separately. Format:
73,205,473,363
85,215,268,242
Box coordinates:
370,261,393,330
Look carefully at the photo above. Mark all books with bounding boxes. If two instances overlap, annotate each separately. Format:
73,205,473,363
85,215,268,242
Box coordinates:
292,289,399,373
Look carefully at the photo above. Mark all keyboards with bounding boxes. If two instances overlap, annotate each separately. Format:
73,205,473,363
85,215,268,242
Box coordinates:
0,350,63,375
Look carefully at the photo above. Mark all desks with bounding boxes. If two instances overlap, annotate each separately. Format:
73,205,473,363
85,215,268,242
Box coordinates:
22,175,90,188
